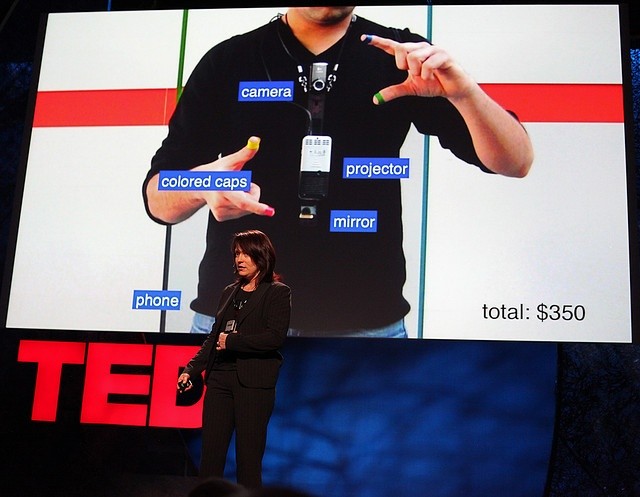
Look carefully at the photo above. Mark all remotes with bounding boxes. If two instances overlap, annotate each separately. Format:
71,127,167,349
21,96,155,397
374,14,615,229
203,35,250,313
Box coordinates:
178,379,192,390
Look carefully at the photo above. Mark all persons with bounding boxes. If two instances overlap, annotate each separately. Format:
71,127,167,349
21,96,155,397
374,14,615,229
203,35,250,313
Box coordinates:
176,230,291,497
142,6,533,338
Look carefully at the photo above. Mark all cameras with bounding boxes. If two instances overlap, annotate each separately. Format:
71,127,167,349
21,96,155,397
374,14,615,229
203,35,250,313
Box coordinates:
307,62,329,95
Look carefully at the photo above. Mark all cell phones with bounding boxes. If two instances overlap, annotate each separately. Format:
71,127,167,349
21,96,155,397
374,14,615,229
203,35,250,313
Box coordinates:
296,134,332,201
225,319,237,334
